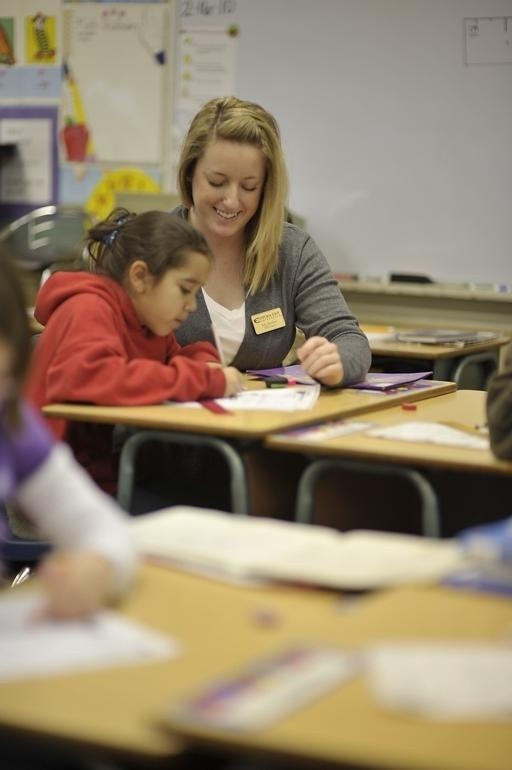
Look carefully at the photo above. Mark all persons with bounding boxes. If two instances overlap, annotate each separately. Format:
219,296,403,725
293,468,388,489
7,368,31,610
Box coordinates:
486,344,512,459
1,256,139,617
164,97,373,389
27,208,244,492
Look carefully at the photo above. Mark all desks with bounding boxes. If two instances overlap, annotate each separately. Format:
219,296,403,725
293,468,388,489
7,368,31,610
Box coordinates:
42,369,458,519
2,504,427,758
162,559,509,769
265,389,511,536
355,325,511,388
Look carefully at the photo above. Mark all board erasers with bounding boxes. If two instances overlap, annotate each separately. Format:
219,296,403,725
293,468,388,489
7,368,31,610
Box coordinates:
390,274,432,284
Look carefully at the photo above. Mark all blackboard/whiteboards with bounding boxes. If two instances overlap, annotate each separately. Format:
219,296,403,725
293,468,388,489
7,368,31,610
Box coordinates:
160,0,512,302
